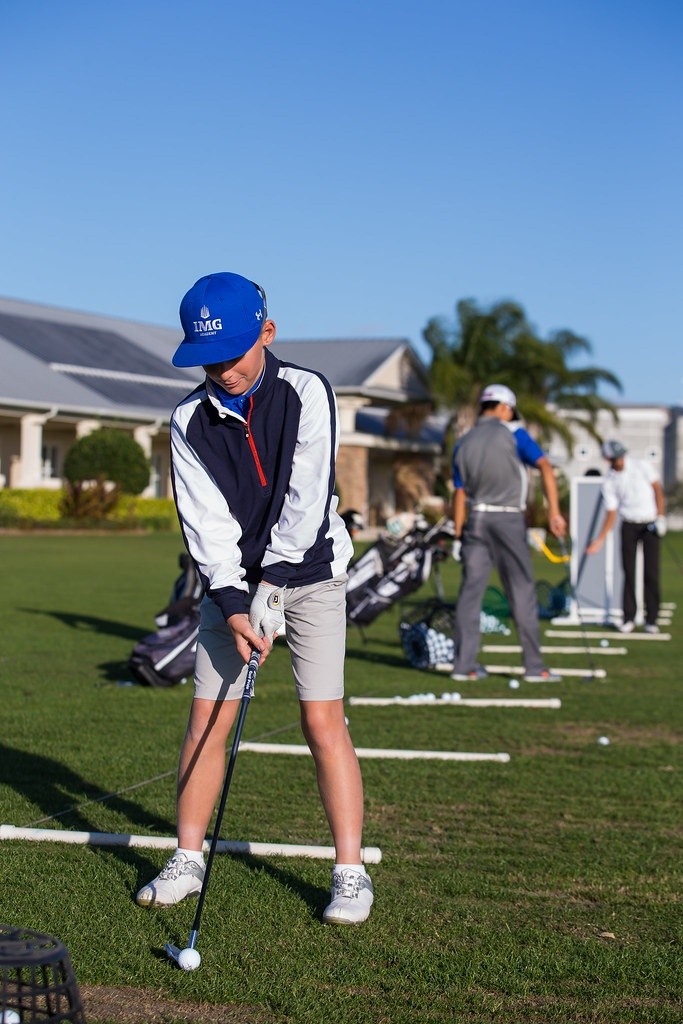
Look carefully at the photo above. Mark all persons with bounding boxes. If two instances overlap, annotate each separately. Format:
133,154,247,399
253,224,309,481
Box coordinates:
450,379,570,687
130,268,379,928
584,439,667,635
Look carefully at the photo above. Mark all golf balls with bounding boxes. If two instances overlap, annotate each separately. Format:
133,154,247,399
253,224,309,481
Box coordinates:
344,604,512,729
178,947,202,970
509,678,520,687
597,734,610,747
600,638,609,648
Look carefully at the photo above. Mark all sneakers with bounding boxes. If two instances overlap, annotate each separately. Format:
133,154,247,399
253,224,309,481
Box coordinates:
322,868,375,924
524,669,563,682
451,669,489,681
136,853,207,908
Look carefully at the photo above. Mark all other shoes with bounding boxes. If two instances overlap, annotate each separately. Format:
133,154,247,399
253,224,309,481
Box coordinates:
620,620,635,632
644,624,661,633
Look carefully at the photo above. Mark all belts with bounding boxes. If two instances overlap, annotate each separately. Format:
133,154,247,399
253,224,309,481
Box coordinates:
470,503,521,512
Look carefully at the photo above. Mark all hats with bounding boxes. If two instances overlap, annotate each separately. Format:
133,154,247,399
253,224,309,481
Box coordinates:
601,439,627,459
480,384,523,420
171,272,267,368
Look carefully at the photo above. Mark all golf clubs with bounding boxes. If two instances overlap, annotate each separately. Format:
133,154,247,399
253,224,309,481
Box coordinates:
558,535,596,680
161,643,263,964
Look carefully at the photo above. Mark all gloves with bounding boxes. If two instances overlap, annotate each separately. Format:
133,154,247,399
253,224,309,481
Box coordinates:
248,583,284,645
452,540,462,561
654,515,668,537
